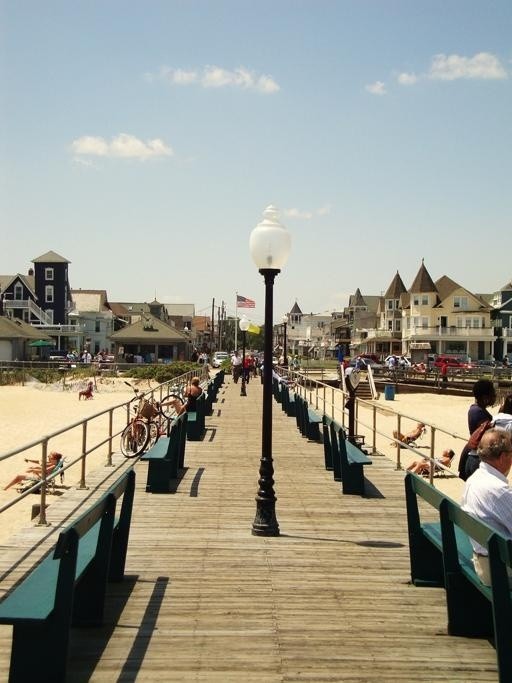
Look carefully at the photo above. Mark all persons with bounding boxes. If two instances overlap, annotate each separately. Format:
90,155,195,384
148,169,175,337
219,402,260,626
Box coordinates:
458,393,512,481
390,421,426,448
459,427,512,590
467,378,497,436
405,448,455,474
1,449,62,490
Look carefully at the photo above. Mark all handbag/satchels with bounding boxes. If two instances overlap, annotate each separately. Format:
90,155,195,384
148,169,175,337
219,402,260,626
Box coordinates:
463,420,494,450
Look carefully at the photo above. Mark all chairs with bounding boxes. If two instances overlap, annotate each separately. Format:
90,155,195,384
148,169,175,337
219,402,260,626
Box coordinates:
418,450,454,477
398,425,429,448
12,454,68,494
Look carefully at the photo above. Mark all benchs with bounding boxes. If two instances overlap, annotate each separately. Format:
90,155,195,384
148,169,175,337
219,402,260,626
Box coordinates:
271,372,374,496
0,466,137,682
402,469,511,683
139,369,227,491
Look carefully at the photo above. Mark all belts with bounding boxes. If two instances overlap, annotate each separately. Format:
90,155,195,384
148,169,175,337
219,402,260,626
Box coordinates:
472,550,488,557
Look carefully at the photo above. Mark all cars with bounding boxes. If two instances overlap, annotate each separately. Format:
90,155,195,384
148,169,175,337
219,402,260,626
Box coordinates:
47,350,67,359
212,349,280,369
349,351,512,378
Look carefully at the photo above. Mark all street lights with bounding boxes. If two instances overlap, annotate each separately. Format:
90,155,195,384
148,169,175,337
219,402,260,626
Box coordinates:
238,313,250,396
247,199,295,538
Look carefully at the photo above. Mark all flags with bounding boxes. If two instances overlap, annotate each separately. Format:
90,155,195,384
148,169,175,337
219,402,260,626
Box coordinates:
247,321,261,333
29,338,55,358
238,295,257,307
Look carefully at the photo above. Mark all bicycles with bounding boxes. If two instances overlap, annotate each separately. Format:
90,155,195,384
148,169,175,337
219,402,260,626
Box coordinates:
116,376,186,460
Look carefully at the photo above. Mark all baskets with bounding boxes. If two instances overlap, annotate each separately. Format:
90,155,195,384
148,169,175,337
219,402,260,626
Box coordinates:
138,395,158,420
